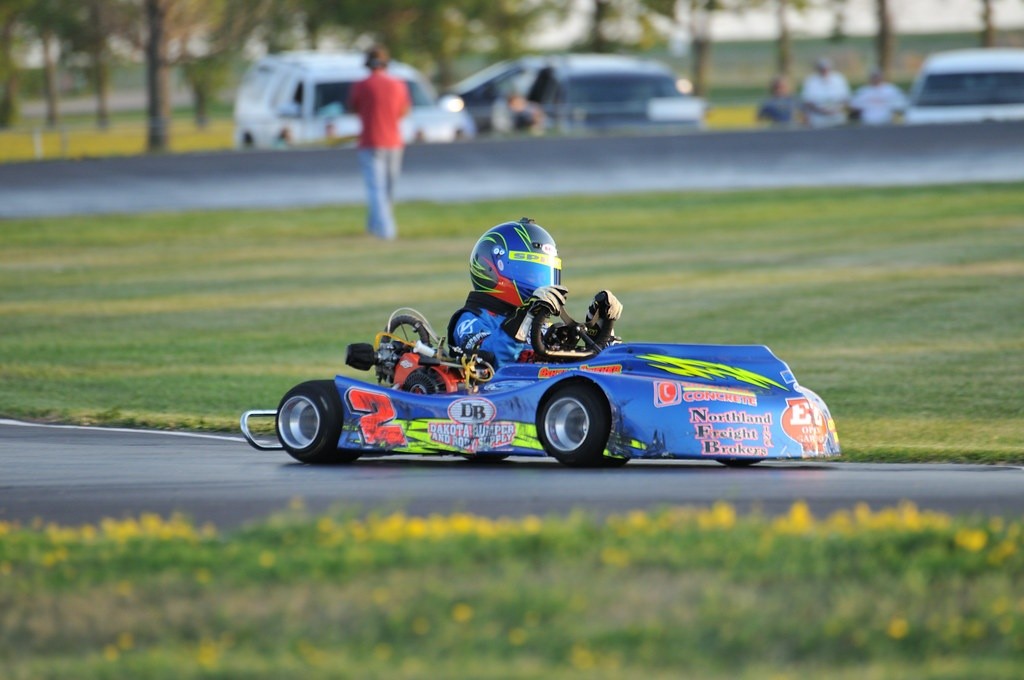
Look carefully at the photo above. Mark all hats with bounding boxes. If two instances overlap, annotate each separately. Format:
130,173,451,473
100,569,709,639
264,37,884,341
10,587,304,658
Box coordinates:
365,47,388,66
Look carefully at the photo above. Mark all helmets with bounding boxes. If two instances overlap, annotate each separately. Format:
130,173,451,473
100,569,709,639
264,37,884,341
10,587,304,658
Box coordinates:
469,217,561,309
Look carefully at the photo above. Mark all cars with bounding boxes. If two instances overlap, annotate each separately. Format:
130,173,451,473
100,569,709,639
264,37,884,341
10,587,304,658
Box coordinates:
234,49,478,157
893,44,1024,125
448,50,705,136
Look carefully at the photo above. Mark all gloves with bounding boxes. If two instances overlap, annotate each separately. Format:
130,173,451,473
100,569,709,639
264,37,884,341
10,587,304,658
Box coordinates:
526,285,569,317
586,289,622,326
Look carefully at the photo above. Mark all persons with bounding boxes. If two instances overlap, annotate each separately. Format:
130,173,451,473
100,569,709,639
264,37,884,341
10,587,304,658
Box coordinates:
351,47,412,242
448,218,623,369
502,92,548,135
758,56,912,127
